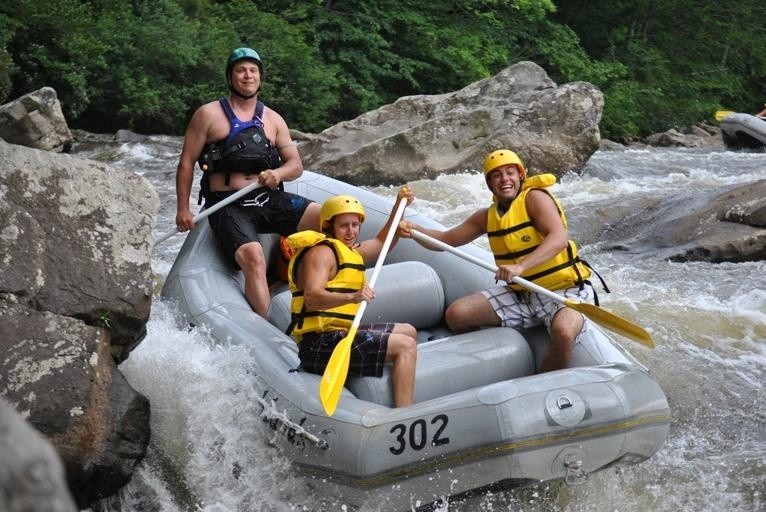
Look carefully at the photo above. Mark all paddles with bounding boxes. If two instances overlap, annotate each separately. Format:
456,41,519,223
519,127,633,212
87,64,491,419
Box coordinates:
716,111,766,121
320,186,409,417
407,229,655,348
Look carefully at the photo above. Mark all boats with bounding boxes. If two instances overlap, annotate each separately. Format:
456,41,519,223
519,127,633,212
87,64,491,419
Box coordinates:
160,171,672,512
719,112,766,151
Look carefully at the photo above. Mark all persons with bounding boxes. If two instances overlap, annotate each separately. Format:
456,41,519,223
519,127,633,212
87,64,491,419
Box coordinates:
393,149,601,379
284,184,418,409
176,47,324,320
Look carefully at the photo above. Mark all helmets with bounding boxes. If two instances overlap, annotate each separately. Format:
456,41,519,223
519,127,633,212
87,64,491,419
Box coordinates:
483,149,526,191
319,194,365,232
226,47,262,72
